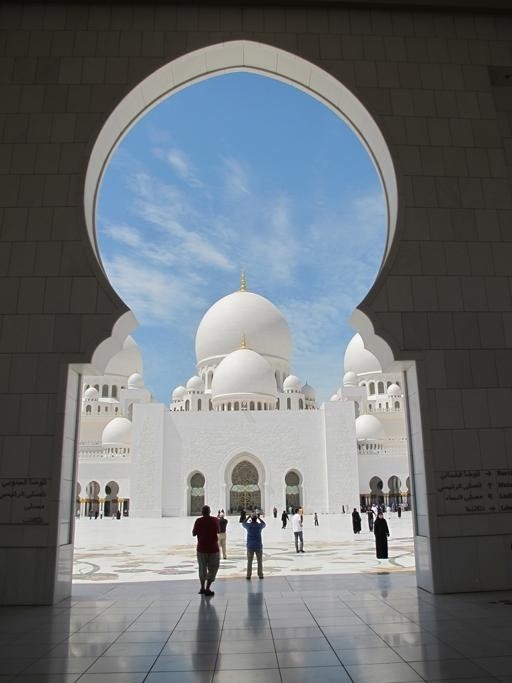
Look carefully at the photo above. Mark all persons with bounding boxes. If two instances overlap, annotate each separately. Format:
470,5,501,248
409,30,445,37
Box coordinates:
88,508,121,520
191,504,219,595
363,503,402,523
375,511,390,559
216,508,228,559
191,592,222,682
292,508,305,553
314,511,319,526
377,559,392,598
365,505,377,530
238,506,247,522
352,507,362,534
241,511,266,580
298,505,305,525
244,578,267,638
281,510,289,528
273,504,278,517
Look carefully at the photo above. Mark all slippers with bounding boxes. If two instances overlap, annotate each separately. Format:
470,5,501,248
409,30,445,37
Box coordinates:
199,590,205,593
206,590,214,595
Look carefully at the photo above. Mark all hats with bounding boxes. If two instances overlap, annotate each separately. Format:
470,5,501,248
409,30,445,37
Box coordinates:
219,512,224,516
251,513,257,518
